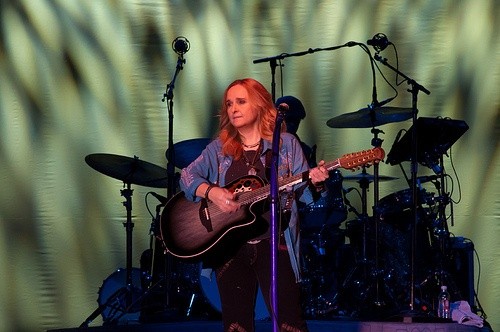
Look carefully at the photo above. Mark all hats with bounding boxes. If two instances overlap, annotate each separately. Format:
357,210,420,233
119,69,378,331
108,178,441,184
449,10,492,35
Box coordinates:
276,96,306,119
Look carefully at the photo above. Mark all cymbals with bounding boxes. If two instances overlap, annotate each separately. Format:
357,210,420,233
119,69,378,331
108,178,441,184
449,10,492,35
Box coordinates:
340,173,397,182
166,139,215,168
327,107,419,128
85,154,180,188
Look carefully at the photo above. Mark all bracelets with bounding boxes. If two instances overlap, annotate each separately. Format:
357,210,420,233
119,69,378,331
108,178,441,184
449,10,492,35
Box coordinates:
205,184,219,203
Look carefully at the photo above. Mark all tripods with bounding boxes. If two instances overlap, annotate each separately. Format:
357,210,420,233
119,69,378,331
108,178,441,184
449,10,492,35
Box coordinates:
300,181,469,323
80,182,167,326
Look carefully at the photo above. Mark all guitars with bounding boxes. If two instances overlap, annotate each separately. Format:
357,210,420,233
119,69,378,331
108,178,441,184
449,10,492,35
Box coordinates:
160,145,386,258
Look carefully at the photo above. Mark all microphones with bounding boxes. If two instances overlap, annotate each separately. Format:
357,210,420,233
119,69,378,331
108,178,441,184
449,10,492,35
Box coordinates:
171,36,190,69
367,34,392,52
378,98,393,106
385,131,402,164
276,102,290,122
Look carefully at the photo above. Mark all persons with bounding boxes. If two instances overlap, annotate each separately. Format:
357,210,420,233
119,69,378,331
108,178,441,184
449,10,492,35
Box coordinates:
180,78,331,332
275,96,317,170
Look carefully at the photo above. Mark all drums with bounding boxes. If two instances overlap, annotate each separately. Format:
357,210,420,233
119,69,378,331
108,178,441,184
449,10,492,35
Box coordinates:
347,216,395,265
376,188,438,236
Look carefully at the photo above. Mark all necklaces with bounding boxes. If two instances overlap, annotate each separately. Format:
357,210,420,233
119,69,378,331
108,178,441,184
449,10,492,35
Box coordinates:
241,141,261,176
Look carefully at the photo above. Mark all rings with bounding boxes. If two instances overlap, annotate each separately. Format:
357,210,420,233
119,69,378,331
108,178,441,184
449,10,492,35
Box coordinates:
226,199,230,204
